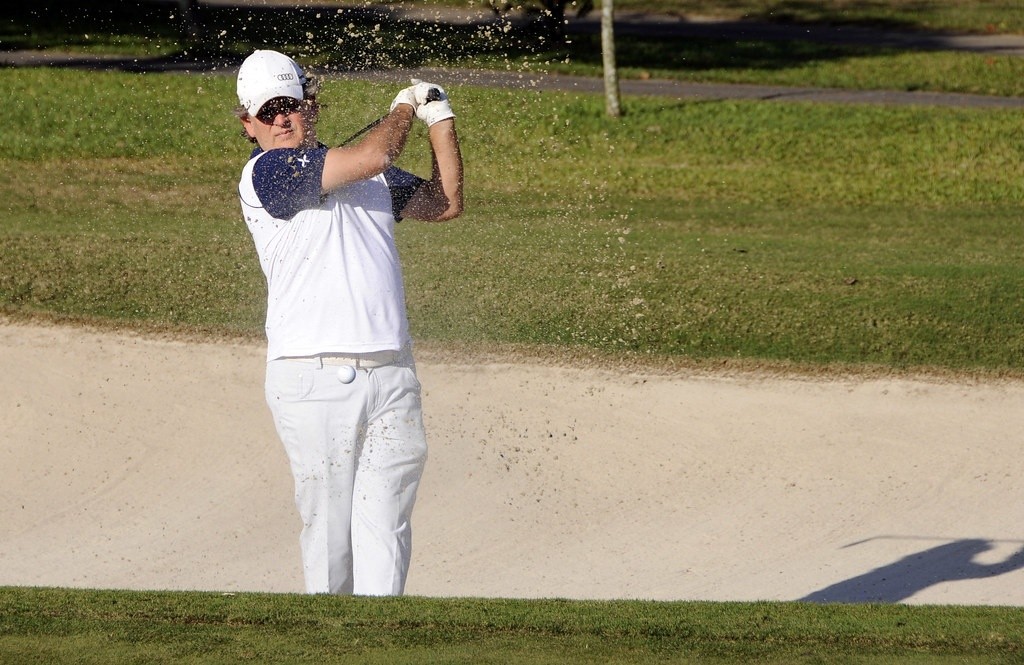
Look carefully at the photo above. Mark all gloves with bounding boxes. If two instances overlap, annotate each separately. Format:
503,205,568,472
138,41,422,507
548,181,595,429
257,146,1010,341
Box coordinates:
410,76,457,127
389,85,418,115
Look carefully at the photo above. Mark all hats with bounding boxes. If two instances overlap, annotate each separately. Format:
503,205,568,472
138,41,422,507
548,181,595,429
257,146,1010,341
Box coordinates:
235,49,309,117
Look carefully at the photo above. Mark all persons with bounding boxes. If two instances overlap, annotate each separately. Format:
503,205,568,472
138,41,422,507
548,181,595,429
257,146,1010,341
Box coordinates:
231,47,468,595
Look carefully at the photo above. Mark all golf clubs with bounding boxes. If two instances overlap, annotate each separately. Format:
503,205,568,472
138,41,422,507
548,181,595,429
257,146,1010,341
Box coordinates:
332,86,445,150
